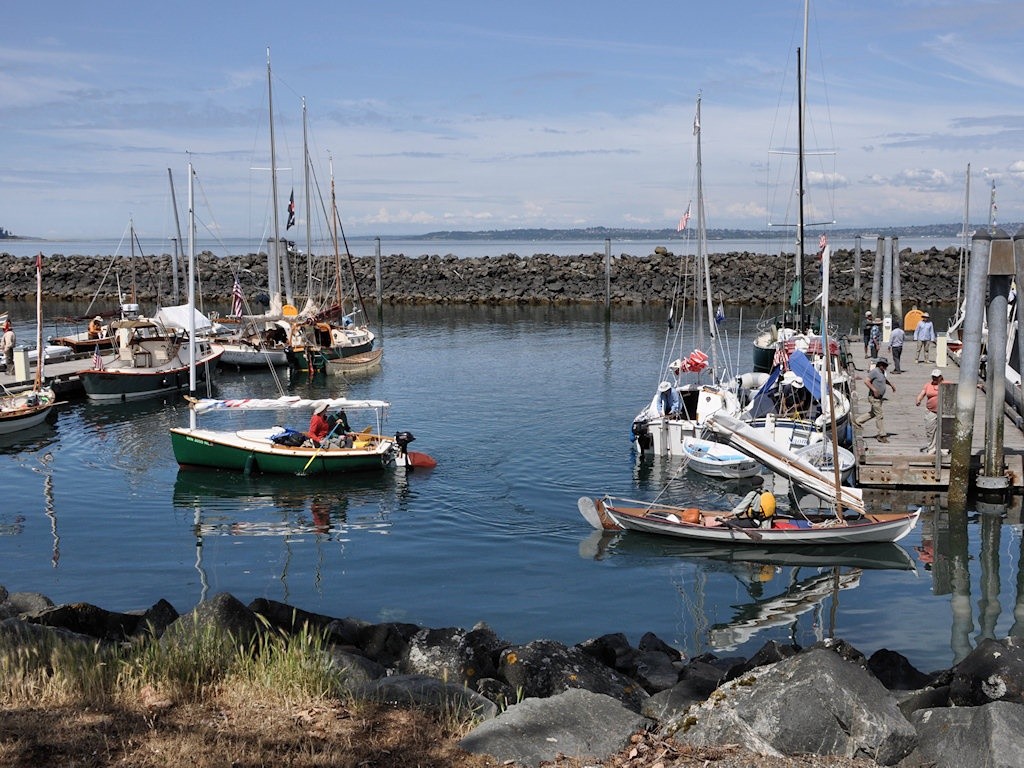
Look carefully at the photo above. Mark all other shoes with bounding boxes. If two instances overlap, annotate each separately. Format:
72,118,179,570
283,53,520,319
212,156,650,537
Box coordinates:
877,436,890,443
852,421,864,430
890,370,901,374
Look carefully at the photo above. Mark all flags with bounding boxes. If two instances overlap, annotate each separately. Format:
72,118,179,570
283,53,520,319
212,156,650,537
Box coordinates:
790,275,803,308
716,303,726,326
232,279,243,319
819,233,826,249
676,203,690,234
93,344,105,371
666,297,677,332
0,311,9,329
35,255,41,272
286,187,296,230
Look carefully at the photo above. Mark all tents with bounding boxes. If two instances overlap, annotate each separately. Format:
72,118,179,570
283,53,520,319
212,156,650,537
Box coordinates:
154,303,213,339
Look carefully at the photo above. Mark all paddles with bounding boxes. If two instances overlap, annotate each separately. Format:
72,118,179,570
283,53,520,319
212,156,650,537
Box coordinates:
715,517,762,541
302,420,342,472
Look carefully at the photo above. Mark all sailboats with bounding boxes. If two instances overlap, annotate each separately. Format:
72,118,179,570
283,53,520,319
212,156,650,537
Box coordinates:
1,46,387,440
579,0,923,550
169,161,438,481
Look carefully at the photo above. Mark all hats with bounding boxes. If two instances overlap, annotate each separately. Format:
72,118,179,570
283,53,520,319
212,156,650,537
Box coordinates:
751,475,764,485
94,316,103,321
877,357,892,365
658,382,672,392
872,318,883,324
893,321,900,328
311,401,331,415
780,371,805,389
921,312,931,318
930,369,942,376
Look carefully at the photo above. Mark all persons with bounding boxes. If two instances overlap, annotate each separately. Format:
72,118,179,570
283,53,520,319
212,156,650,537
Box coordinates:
87,315,103,340
308,401,356,448
852,357,897,443
913,311,935,364
742,561,774,598
916,369,951,454
657,381,683,417
887,321,906,375
914,510,935,573
780,371,807,413
0,318,16,376
714,475,766,528
863,310,883,359
